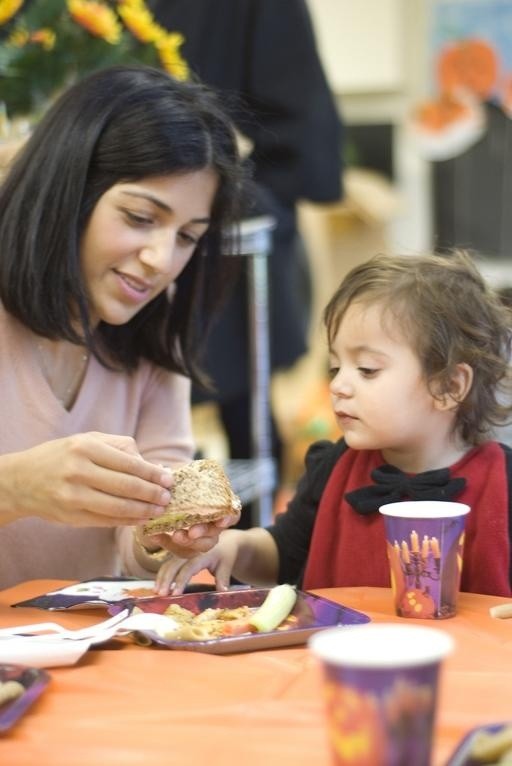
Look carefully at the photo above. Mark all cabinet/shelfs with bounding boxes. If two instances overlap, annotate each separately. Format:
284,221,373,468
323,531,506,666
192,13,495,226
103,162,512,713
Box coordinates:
184,209,282,529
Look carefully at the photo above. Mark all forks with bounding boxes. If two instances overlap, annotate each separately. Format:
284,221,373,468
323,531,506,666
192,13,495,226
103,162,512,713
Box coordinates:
0,607,129,641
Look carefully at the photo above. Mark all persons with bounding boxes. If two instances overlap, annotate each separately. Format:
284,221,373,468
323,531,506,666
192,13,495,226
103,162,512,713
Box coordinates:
152,249,512,600
142,0,347,588
0,65,243,586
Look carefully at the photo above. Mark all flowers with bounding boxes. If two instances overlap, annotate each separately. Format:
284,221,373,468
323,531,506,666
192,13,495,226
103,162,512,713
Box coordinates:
0,0,189,119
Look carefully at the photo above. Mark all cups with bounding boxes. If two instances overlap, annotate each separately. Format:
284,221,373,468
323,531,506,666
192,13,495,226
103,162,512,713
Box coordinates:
377,499,472,619
307,622,451,765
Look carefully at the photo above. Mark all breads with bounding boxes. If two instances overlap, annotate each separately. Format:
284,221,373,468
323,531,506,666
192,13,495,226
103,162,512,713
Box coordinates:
141,458,242,535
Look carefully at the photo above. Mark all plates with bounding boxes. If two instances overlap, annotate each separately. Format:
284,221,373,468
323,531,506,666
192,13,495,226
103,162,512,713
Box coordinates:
107,586,374,658
